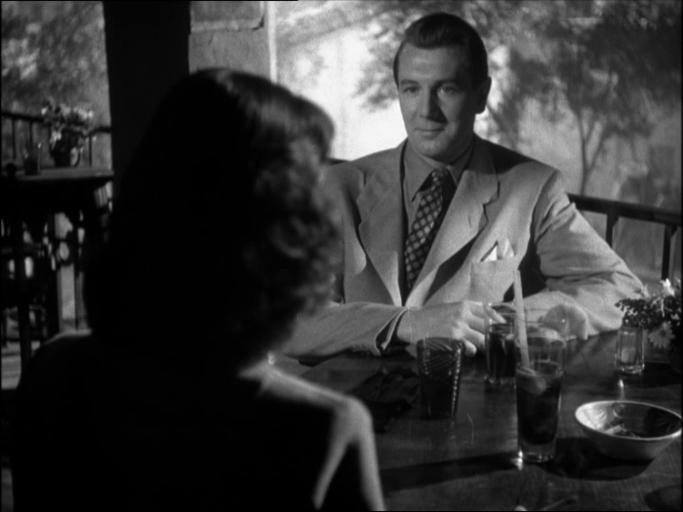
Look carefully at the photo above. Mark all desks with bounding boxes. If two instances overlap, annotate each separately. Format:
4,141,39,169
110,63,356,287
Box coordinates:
0,164,114,370
298,348,681,512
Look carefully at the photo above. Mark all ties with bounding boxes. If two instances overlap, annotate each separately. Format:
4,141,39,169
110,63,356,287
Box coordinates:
403,169,450,291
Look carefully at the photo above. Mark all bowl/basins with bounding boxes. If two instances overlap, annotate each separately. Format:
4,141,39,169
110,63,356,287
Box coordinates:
574,399,682,464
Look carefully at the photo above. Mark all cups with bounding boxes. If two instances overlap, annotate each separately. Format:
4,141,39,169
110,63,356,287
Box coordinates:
512,307,567,464
416,336,465,430
22,139,43,176
483,303,516,386
613,326,645,374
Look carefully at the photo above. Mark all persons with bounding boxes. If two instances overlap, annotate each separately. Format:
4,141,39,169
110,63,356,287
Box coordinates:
12,66,395,512
267,13,647,361
